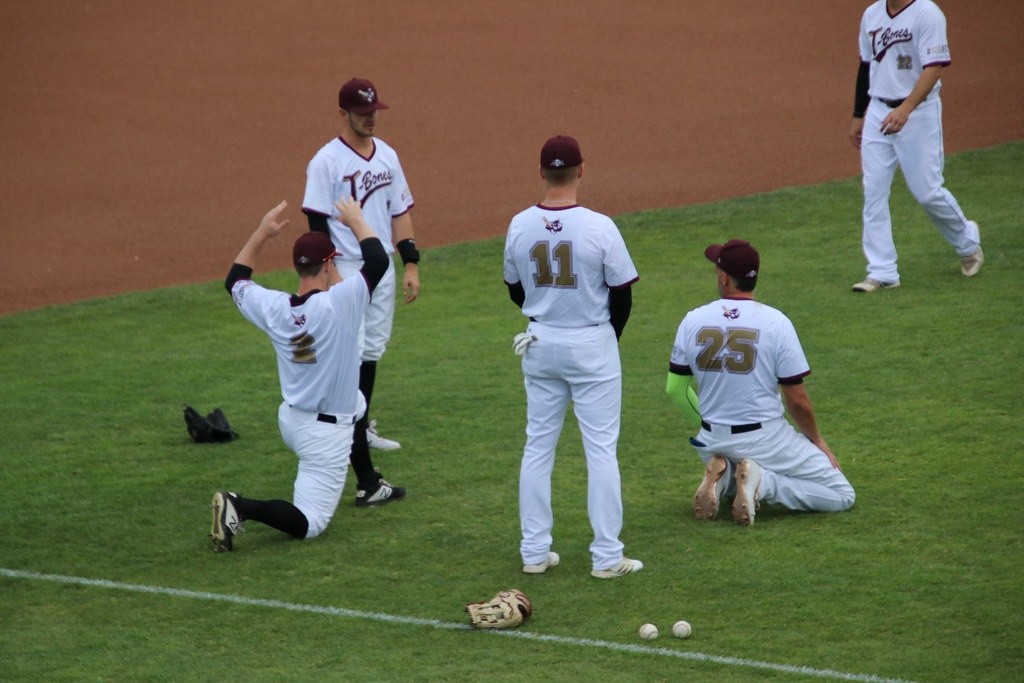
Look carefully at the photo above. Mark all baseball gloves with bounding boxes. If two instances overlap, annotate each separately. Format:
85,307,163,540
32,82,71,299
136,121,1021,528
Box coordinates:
181,405,240,444
463,587,533,631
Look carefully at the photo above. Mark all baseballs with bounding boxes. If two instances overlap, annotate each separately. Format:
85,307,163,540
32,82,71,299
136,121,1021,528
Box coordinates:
638,624,659,640
671,621,692,639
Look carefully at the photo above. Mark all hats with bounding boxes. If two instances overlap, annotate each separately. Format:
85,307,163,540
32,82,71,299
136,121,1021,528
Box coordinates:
703,239,760,278
338,77,389,113
292,231,344,267
540,135,586,169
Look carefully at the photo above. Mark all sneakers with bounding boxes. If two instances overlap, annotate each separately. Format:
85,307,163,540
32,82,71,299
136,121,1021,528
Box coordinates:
523,552,560,574
209,491,246,553
591,555,644,579
355,472,407,508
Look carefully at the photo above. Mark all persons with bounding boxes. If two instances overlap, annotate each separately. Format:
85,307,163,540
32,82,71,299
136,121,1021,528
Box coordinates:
667,239,856,527
301,77,421,452
210,194,407,552
849,0,984,292
504,135,642,579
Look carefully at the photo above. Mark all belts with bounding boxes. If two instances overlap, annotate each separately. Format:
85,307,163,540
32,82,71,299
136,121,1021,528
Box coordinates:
701,421,762,434
878,98,906,108
290,404,358,424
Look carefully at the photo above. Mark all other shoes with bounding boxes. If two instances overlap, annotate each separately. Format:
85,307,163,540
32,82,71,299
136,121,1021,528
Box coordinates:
366,419,401,451
692,454,731,521
731,458,760,527
961,221,984,277
851,278,901,292
